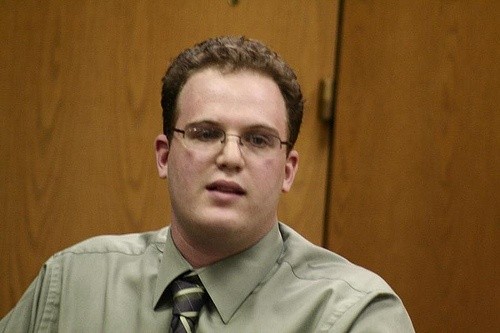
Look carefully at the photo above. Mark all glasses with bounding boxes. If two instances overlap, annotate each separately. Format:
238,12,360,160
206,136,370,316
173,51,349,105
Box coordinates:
168,121,295,158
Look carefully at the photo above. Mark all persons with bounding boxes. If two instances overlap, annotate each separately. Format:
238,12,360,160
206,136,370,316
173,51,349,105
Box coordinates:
0,37,418,332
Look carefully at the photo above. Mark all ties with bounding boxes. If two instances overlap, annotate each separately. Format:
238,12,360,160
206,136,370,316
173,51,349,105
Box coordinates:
164,275,204,333
